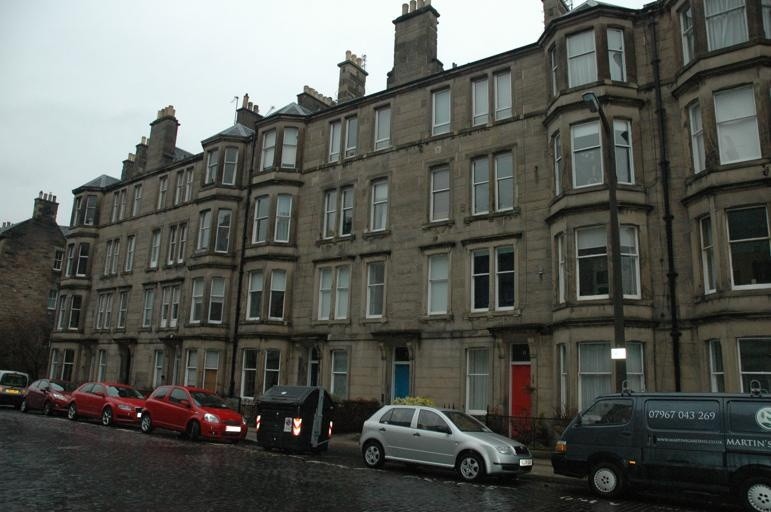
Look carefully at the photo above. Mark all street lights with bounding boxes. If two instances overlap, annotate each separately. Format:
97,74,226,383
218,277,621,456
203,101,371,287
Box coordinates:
583,89,627,395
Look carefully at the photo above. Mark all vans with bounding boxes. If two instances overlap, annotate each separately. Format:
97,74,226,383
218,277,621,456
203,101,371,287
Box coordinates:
552,392,771,512
0,370,29,409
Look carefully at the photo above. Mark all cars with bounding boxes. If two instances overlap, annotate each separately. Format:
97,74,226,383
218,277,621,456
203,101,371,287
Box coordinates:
67,382,147,427
20,378,72,415
139,385,248,445
360,404,534,484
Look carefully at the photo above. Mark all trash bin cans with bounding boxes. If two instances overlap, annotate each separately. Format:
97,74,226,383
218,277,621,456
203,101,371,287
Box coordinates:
254,385,336,455
